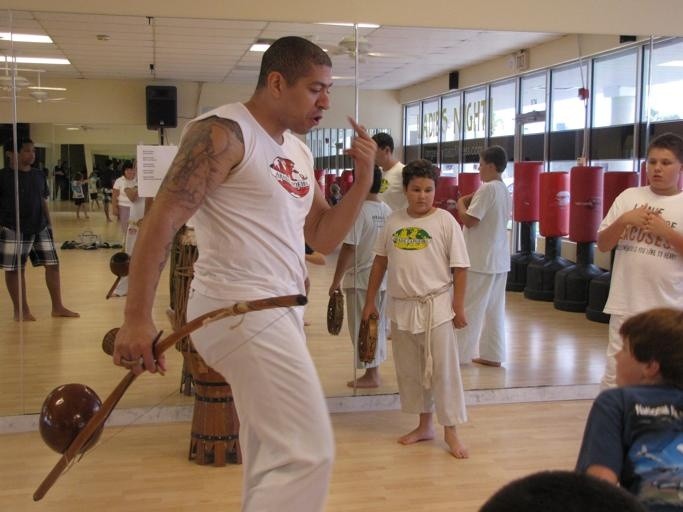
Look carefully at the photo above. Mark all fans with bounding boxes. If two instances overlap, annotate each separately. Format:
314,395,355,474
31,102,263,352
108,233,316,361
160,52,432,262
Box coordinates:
0,48,67,104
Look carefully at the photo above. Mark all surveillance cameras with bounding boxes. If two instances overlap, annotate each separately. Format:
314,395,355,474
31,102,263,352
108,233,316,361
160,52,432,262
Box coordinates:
149,63,155,70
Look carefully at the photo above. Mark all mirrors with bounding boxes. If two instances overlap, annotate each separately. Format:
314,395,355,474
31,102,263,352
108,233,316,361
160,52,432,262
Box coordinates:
306,128,391,204
33,142,157,211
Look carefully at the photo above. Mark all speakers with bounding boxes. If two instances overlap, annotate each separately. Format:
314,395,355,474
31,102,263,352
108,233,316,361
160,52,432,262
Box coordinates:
448,72,459,88
145,85,178,130
619,36,636,43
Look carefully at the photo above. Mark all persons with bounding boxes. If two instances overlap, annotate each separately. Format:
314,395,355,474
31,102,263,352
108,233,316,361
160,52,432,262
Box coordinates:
112,36,378,512
454,145,511,367
0,137,80,322
358,159,471,459
478,470,646,512
575,309,683,512
328,163,394,388
596,132,683,389
39,133,410,266
